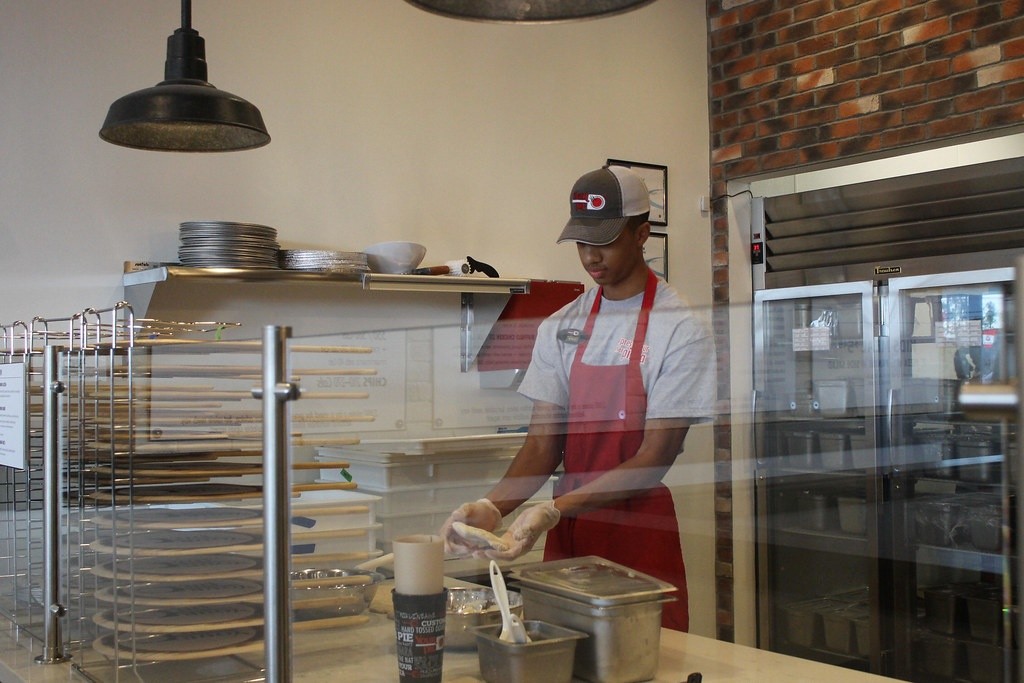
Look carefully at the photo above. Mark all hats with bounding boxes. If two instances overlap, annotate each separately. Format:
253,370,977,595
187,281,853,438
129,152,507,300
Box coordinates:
556,165,651,246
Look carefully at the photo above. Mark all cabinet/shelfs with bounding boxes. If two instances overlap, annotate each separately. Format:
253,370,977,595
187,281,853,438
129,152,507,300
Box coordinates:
1,261,531,682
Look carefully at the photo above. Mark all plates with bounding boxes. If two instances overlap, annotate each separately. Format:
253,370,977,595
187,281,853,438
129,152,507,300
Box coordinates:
280,250,370,271
177,220,281,268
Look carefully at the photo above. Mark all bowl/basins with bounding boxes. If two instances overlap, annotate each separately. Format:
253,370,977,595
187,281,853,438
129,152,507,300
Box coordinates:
289,568,386,617
445,587,522,650
363,242,426,273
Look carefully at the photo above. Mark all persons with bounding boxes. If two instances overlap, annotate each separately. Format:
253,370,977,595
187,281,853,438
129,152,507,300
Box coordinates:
437,164,719,632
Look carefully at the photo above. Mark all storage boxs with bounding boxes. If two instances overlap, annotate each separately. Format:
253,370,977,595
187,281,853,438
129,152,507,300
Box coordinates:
467,622,589,683
505,553,679,681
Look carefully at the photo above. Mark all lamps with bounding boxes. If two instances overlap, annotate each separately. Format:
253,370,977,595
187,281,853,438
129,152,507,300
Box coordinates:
100,0,269,151
